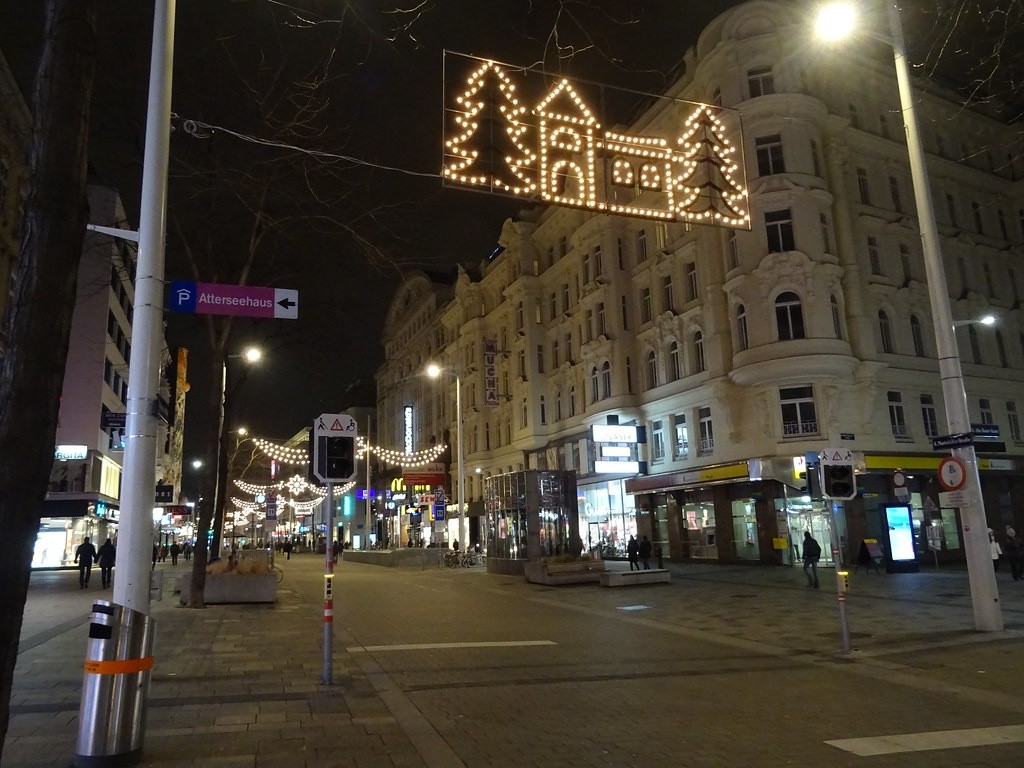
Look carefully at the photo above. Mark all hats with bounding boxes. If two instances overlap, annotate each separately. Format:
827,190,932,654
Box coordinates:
1007,525,1015,535
988,528,994,534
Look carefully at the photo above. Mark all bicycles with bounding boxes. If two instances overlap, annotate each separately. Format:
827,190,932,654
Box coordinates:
437,549,486,569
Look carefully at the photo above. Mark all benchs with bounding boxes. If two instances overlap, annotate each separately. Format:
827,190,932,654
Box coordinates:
599,569,672,587
546,562,611,585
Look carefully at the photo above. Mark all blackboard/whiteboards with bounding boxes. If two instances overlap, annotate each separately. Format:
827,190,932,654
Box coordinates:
856,541,877,566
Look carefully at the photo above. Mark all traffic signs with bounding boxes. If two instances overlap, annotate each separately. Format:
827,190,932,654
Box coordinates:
162,278,299,318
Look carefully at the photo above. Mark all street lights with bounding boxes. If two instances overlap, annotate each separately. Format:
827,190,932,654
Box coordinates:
427,365,466,550
814,1,1003,630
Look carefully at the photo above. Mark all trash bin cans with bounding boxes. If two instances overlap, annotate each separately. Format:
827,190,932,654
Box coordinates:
467,544,476,564
74,599,155,768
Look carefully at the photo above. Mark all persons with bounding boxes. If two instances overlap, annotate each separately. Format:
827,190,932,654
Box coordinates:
656,546,663,569
153,541,196,570
333,540,348,564
803,531,821,588
579,536,585,556
476,537,482,554
791,526,800,560
627,535,652,570
408,538,413,548
453,539,458,551
243,539,263,549
267,537,296,560
74,537,115,589
1005,525,1024,581
988,528,1003,571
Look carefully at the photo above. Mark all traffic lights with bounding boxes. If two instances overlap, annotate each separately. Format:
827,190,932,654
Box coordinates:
824,464,854,497
327,436,348,478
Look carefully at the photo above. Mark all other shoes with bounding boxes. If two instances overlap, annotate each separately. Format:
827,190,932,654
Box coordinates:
813,586,819,589
1013,576,1019,581
85,585,88,588
808,583,814,586
1019,575,1024,580
104,585,106,589
80,586,84,589
107,583,109,587
